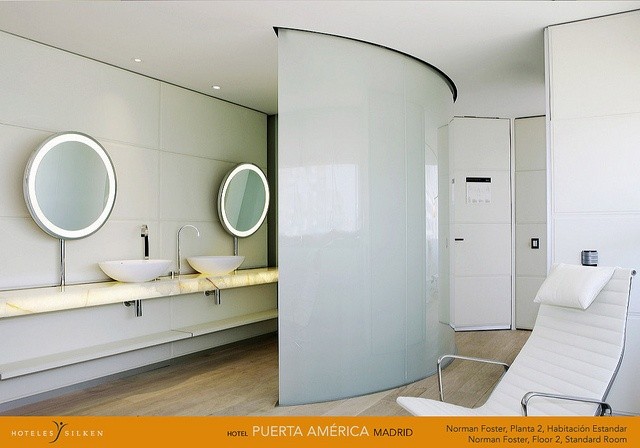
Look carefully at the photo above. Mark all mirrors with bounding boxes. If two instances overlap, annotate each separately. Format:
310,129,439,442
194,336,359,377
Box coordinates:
21,131,118,242
217,162,271,238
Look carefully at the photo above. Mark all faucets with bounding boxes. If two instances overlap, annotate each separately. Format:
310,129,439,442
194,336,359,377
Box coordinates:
141,224,149,257
178,225,199,274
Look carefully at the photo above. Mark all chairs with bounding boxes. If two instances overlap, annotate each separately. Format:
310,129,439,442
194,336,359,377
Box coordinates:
395,267,637,417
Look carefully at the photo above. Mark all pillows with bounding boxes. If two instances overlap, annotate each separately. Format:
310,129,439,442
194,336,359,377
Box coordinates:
533,263,616,311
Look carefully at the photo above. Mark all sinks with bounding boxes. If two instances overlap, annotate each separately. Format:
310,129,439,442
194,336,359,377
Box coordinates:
99,260,173,282
186,255,244,273
